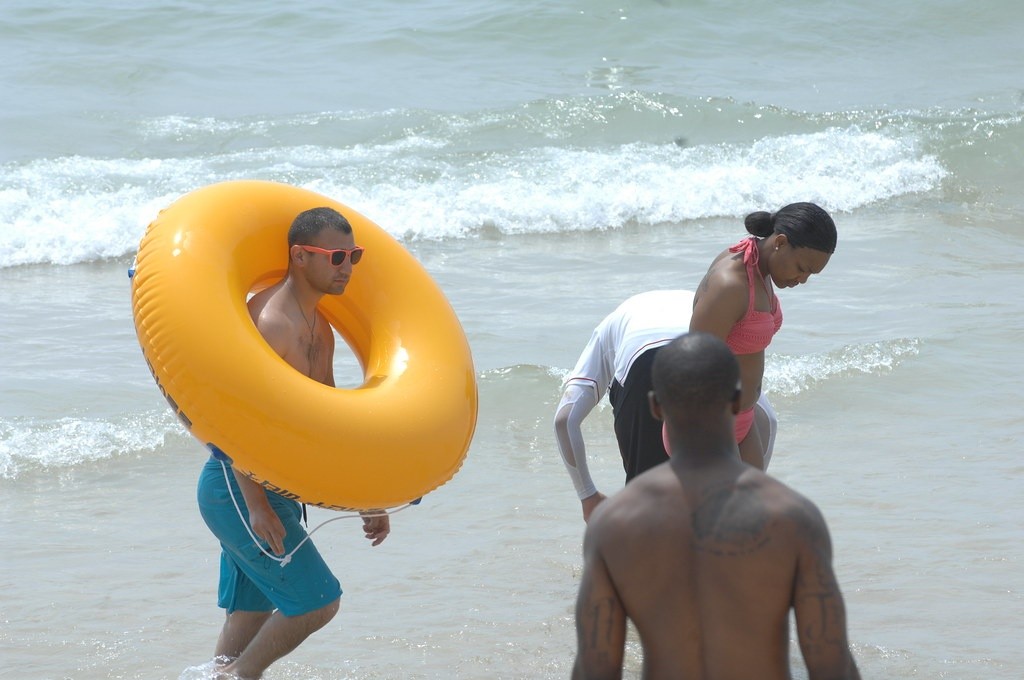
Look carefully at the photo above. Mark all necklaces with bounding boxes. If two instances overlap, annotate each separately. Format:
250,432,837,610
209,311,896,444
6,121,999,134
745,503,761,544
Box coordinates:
295,284,315,342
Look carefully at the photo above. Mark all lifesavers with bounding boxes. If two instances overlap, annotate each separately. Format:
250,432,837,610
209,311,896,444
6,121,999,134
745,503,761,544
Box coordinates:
130,182,479,513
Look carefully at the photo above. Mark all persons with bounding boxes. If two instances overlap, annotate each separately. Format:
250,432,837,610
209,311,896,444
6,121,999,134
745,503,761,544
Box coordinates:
553,288,697,524
571,333,864,679
194,205,391,680
731,378,778,476
685,200,839,476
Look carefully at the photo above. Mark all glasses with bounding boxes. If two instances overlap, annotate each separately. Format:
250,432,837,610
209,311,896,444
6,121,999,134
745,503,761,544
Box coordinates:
300,245,365,267
